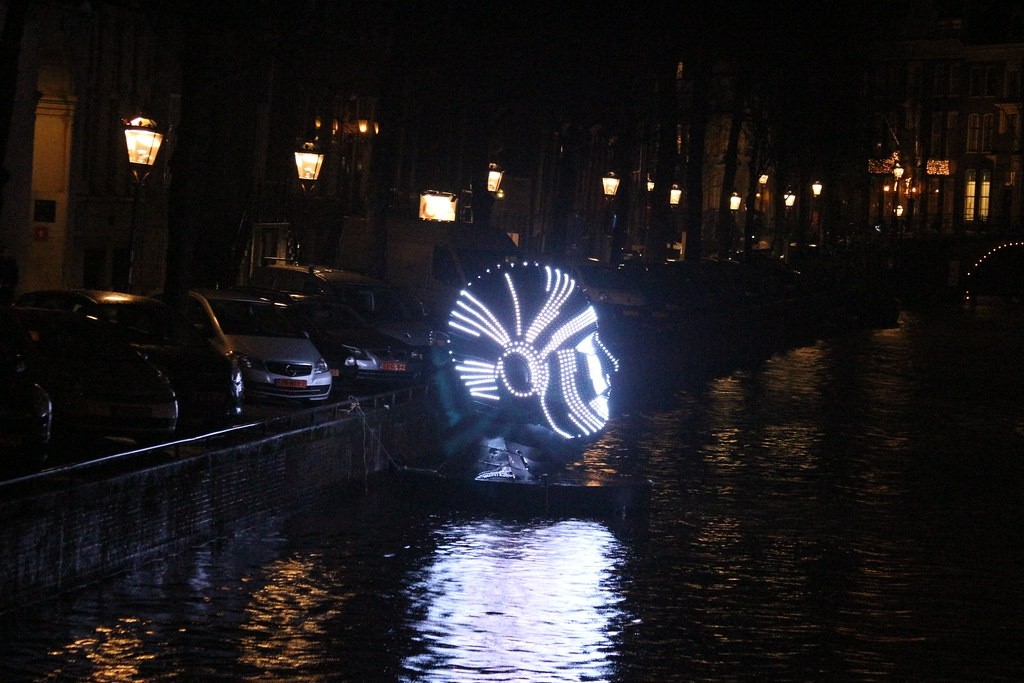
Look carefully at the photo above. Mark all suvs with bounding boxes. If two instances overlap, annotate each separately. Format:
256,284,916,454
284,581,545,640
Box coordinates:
17,291,239,428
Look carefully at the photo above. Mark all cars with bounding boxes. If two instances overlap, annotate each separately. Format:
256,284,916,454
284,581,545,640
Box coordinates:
246,291,421,397
0,306,178,441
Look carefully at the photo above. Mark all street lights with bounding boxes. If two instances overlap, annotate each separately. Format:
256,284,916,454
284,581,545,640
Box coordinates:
784,190,794,248
599,168,623,261
474,158,503,254
810,181,823,238
113,108,170,293
727,191,740,254
668,183,681,264
295,136,326,297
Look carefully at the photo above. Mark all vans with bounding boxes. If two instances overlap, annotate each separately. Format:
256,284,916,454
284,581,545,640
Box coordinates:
173,287,332,406
263,262,448,374
